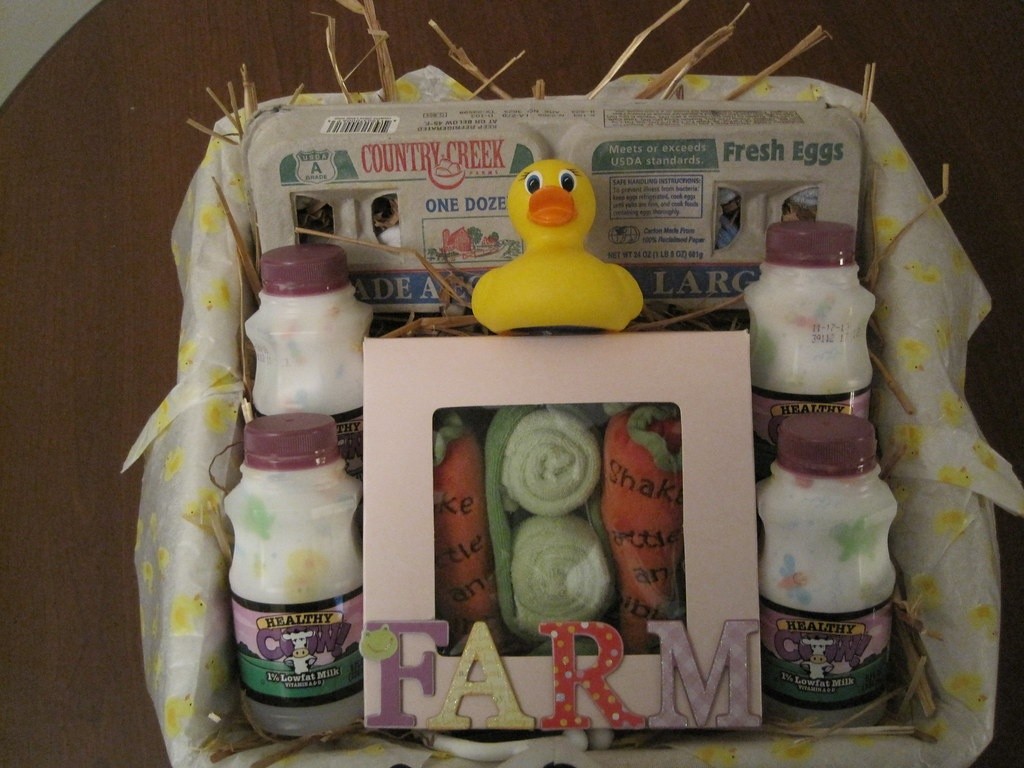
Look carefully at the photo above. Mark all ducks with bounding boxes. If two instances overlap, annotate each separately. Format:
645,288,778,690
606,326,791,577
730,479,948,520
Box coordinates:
471,157,645,335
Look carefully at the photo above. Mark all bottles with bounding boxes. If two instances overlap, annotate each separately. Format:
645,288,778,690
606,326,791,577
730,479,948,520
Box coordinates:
225,412,364,741
756,413,894,728
242,243,373,508
743,221,876,448
